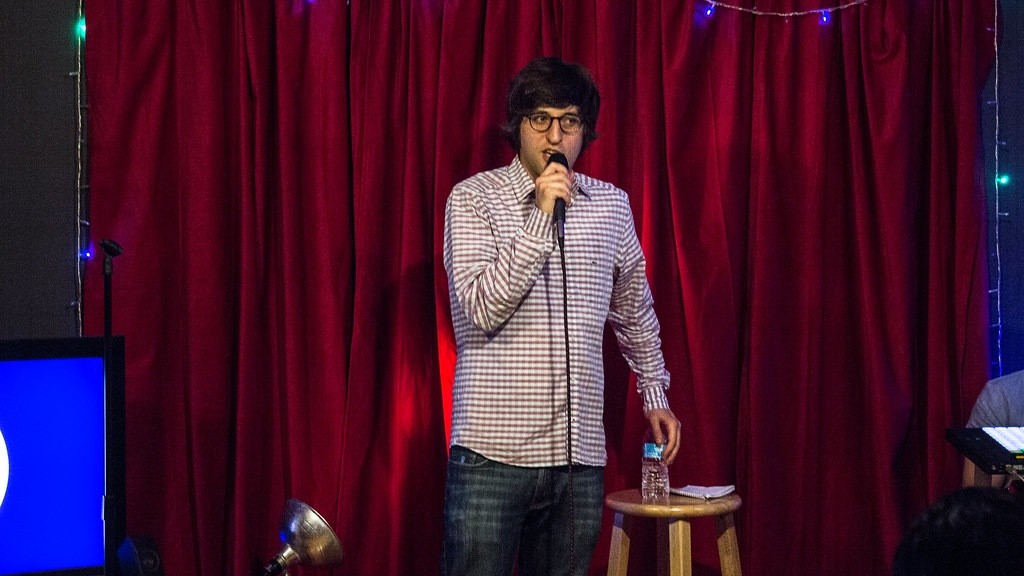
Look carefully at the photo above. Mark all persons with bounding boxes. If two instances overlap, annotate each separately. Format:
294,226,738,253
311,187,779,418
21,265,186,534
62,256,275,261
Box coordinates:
890,364,1024,576
442,58,681,576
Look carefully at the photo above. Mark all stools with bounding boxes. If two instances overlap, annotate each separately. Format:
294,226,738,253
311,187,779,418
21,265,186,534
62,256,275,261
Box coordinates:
603,489,743,576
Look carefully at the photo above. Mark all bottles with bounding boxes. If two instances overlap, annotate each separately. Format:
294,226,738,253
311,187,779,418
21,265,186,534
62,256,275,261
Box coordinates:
642,425,670,499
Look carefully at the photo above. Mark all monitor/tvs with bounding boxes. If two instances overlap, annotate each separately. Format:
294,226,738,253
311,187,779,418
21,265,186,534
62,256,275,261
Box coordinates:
0,333,125,576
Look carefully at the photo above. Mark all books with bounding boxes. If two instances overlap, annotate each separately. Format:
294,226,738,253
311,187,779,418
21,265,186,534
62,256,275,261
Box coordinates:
671,485,736,500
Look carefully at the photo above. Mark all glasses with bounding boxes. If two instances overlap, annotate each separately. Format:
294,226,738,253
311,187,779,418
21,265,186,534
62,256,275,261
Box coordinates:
523,111,585,134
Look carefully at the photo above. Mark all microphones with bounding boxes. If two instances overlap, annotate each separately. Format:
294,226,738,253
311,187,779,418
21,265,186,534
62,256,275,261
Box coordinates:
546,152,569,250
